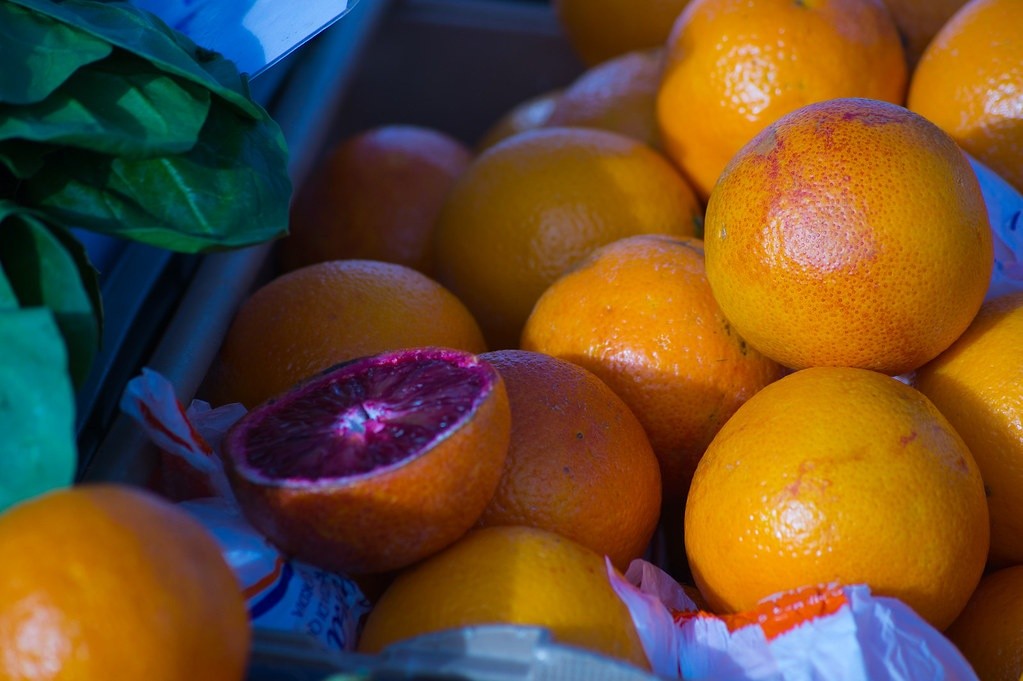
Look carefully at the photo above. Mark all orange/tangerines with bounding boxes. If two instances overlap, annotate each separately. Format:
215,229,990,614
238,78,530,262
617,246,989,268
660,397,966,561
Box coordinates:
2,0,1022,681
